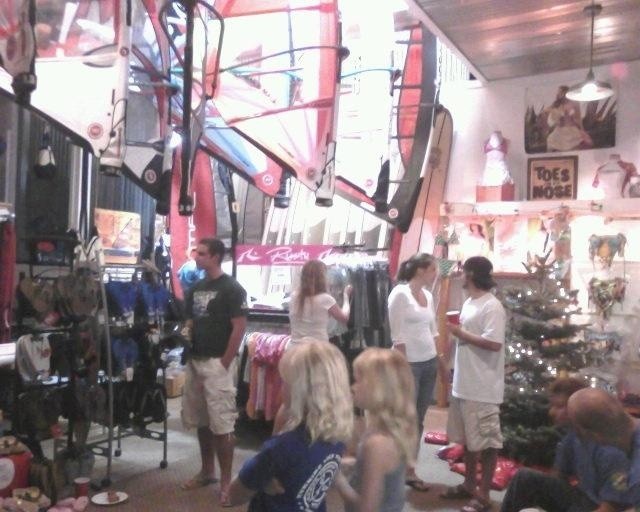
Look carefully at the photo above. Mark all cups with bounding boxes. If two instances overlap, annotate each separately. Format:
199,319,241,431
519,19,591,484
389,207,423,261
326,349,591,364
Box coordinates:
446,311,460,326
73,476,91,496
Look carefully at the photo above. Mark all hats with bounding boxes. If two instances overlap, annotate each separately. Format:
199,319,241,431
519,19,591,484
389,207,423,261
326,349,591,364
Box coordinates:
461,254,499,292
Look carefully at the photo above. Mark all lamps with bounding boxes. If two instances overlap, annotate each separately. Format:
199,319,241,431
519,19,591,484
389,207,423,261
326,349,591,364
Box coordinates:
33,120,58,181
565,3,614,105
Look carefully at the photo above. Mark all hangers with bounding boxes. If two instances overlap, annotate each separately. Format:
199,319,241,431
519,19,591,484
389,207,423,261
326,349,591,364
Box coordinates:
315,242,391,273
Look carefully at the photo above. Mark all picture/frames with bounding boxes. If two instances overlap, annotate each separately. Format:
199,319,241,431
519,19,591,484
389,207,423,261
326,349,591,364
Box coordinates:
525,154,578,200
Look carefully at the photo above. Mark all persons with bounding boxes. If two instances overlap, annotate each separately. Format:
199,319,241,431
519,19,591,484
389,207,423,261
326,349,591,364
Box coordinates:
481,131,510,184
567,386,639,512
387,253,450,493
499,378,630,512
438,257,507,512
594,154,638,199
273,259,353,436
179,237,249,507
332,347,418,512
227,340,354,512
469,223,485,239
546,86,593,152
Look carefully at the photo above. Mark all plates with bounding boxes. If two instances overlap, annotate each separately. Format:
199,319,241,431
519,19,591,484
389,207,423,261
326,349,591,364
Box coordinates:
90,491,128,506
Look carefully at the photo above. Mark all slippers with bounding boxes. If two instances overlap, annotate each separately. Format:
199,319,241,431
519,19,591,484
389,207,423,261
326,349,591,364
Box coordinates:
180,472,220,491
7,268,174,497
218,484,235,507
438,483,478,499
406,473,432,491
461,493,492,512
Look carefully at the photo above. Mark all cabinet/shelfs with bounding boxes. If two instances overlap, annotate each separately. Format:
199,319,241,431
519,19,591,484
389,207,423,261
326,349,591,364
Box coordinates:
434,190,638,417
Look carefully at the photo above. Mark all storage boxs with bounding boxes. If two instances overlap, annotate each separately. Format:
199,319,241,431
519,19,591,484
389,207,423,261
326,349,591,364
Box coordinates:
164,374,185,399
